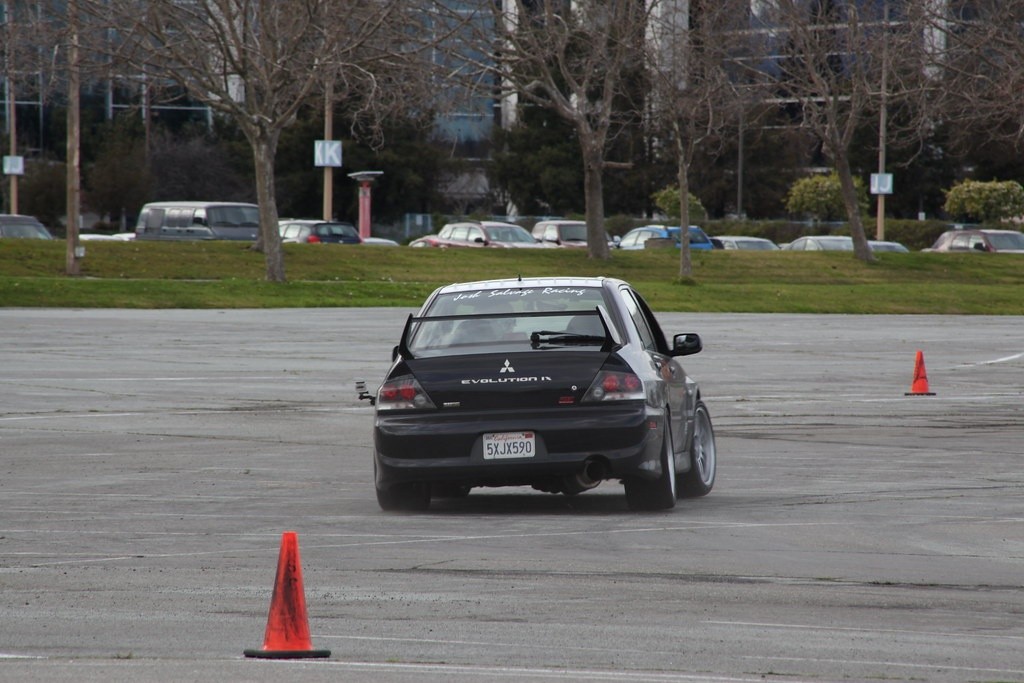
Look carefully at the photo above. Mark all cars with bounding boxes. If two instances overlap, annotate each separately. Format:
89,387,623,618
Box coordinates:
0,214,54,239
116,233,136,240
372,278,716,511
362,237,398,245
531,220,622,249
781,235,855,252
868,241,909,252
409,235,438,248
922,229,1024,254
709,236,781,251
78,234,125,241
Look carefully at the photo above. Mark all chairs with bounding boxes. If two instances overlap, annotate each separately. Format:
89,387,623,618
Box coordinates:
452,321,504,343
568,314,605,336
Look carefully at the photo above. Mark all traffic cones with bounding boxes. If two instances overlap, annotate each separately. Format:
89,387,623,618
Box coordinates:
903,352,937,395
242,531,333,659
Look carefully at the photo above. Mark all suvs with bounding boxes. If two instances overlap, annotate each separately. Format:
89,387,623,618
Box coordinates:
609,223,713,249
438,219,558,249
249,217,362,245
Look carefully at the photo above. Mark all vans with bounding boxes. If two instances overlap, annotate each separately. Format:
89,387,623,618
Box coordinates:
134,200,264,242
